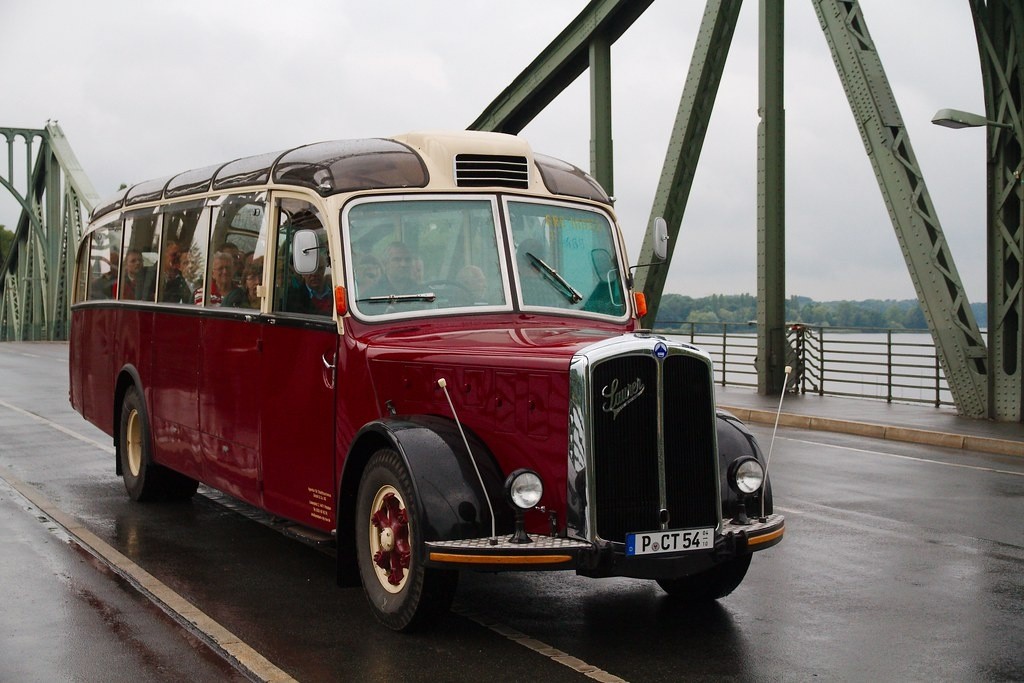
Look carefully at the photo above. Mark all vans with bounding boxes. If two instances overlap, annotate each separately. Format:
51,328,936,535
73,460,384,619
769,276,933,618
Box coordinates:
69,129,792,629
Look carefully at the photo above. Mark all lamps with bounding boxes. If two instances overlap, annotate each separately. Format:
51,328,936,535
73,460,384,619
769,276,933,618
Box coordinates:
930,108,1013,134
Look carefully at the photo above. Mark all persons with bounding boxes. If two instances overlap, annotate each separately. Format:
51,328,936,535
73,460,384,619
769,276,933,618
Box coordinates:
88,240,332,318
354,239,577,316
